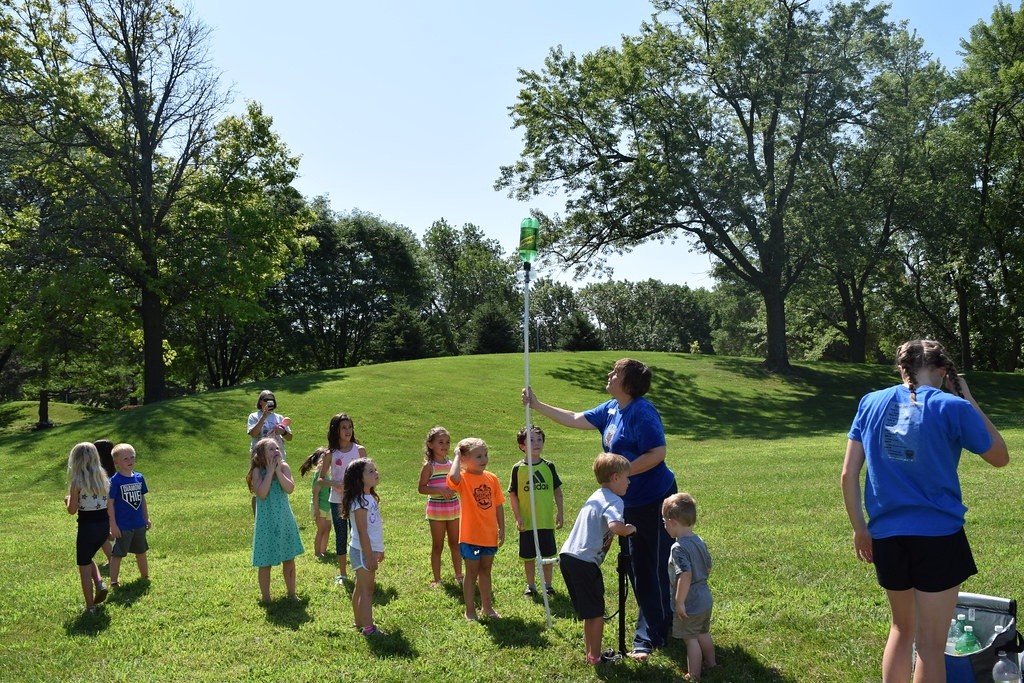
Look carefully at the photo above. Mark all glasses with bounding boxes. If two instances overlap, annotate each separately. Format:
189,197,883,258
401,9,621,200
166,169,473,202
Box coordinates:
263,399,276,402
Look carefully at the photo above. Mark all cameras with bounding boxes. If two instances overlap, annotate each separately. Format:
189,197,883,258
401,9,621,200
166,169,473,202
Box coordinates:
267,401,274,412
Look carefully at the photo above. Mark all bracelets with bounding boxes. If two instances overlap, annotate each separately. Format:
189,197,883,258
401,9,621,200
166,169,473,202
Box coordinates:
281,429,287,436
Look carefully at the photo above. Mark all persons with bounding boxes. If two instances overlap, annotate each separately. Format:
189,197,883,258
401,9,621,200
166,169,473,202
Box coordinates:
267,417,291,439
662,493,716,682
317,414,366,587
418,427,467,588
341,457,388,638
559,452,637,665
245,390,292,517
107,443,151,589
93,439,116,563
298,447,333,560
842,337,1009,683
445,437,505,620
509,425,564,596
64,442,111,614
245,438,304,606
522,358,679,661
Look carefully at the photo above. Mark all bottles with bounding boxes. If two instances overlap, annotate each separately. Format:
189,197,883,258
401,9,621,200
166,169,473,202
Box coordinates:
984,625,1004,648
992,650,1022,683
518,217,539,271
944,614,982,656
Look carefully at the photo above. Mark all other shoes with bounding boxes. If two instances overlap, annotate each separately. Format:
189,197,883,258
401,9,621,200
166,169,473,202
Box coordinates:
88,606,96,614
455,577,463,585
545,585,556,595
601,648,615,658
600,654,622,668
93,582,107,604
362,626,389,638
626,650,651,660
524,584,537,598
288,594,303,602
481,610,501,619
430,580,442,588
464,613,478,621
